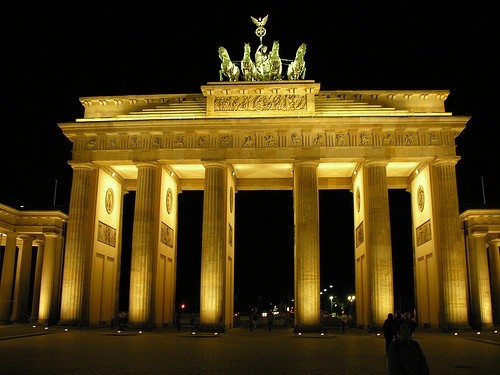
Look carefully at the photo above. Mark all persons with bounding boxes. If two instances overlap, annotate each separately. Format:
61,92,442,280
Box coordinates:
249,317,254,333
176,313,182,331
190,313,197,330
342,321,345,333
387,319,431,375
268,316,273,331
382,311,417,354
